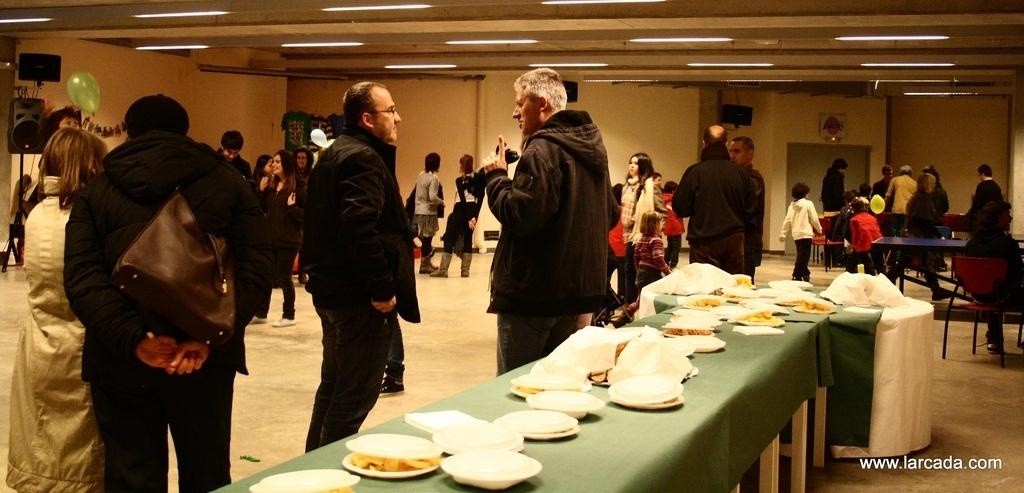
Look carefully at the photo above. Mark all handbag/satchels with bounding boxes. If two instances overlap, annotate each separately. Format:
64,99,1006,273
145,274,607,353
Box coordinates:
110,190,238,350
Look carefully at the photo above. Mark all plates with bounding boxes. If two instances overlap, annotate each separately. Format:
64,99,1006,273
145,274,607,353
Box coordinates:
677,279,837,327
248,410,580,493
511,310,726,418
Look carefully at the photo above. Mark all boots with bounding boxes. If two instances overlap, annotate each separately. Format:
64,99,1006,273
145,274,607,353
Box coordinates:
461,252,472,277
419,254,439,274
377,364,404,396
429,252,452,277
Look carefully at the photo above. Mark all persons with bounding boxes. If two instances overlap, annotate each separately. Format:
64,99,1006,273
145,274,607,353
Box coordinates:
778,182,823,282
378,311,407,397
609,124,766,321
430,153,486,277
299,83,419,453
412,152,447,274
63,94,276,492
49,104,82,130
481,68,622,377
6,128,111,493
819,158,1020,353
213,130,323,327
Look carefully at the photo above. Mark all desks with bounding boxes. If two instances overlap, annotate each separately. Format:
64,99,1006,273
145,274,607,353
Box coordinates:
871,237,1024,295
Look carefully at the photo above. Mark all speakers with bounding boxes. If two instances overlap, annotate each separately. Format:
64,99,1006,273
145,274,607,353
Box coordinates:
717,105,754,127
18,51,65,83
11,94,47,153
565,79,578,103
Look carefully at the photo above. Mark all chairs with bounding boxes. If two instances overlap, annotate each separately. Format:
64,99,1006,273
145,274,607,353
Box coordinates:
942,255,1024,367
812,219,843,272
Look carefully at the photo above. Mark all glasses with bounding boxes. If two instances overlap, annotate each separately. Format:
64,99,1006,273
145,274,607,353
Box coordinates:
369,107,397,117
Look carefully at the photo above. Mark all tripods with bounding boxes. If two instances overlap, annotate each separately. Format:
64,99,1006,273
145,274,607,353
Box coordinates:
1,152,32,276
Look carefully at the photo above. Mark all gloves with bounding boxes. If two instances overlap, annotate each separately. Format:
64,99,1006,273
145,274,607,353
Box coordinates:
469,143,519,196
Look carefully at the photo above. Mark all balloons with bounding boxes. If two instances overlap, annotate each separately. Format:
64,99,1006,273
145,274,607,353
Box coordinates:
67,69,100,118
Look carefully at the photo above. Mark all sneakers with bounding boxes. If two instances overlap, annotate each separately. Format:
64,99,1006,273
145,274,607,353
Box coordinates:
248,316,267,324
987,343,999,354
272,317,296,327
932,290,953,301
